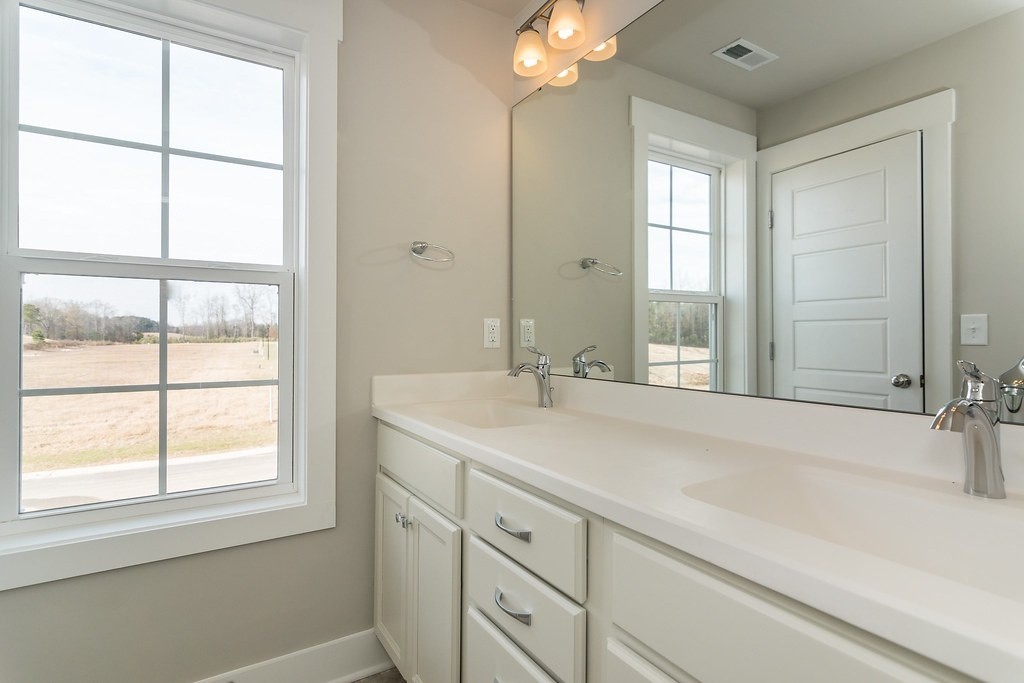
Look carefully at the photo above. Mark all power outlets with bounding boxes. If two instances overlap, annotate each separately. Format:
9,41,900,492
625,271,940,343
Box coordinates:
484,318,500,348
519,320,536,347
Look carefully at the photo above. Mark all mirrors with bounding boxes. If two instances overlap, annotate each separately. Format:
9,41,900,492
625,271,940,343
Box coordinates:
511,0,1024,426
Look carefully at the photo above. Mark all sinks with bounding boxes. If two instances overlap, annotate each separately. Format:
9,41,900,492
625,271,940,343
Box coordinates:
411,398,579,431
681,457,1024,611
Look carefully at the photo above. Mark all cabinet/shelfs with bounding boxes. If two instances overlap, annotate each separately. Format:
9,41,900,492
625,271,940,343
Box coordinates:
373,418,981,683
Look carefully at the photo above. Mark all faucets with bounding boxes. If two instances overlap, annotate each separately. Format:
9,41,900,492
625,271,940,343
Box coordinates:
996,354,1024,427
507,345,554,409
572,345,611,379
929,359,1008,501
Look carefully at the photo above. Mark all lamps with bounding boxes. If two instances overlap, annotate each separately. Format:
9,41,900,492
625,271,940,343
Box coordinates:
513,0,585,77
548,36,618,87
